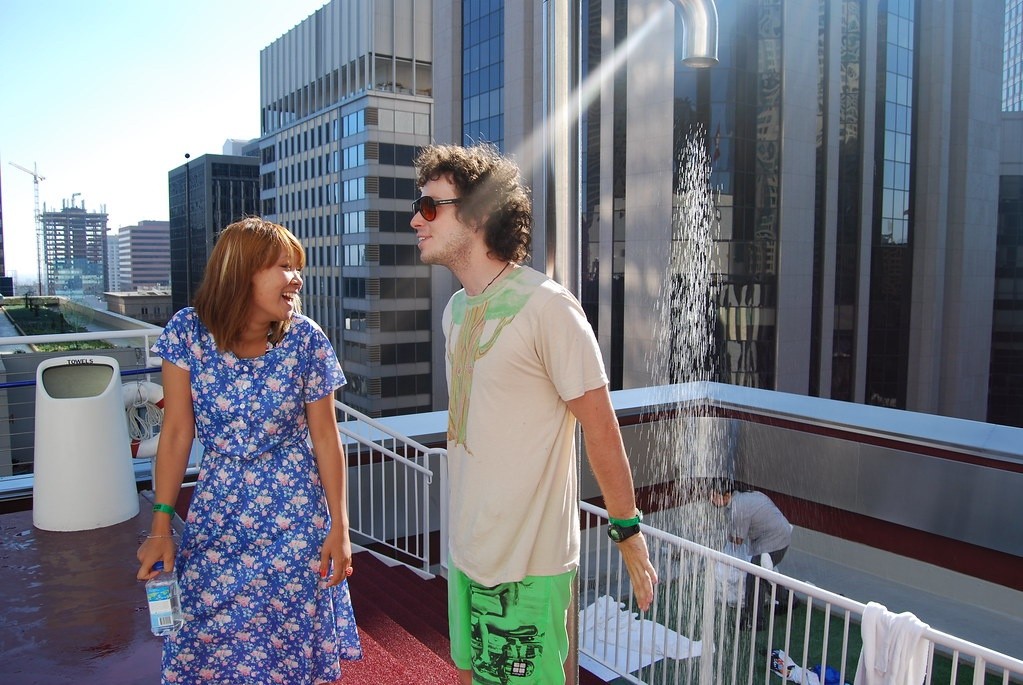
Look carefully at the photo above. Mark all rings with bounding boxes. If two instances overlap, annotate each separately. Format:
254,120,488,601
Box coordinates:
346,567,352,576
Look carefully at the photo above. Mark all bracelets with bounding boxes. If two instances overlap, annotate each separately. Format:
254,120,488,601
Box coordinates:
152,504,174,520
609,508,642,527
147,535,172,538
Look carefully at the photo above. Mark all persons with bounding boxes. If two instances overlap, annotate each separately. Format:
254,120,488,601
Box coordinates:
708,480,798,630
136,218,364,685
411,145,659,684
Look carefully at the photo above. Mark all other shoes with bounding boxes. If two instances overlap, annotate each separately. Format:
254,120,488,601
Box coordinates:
732,619,765,631
769,593,799,615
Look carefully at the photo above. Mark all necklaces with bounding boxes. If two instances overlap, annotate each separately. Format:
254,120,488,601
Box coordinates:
481,262,509,293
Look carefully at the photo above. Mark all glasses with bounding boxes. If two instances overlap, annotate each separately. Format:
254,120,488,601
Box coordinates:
412,196,464,222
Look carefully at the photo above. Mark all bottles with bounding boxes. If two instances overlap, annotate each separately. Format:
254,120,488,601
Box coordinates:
145,561,183,636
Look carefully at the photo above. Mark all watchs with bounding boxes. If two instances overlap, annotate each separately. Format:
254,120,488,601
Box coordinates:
607,524,640,543
